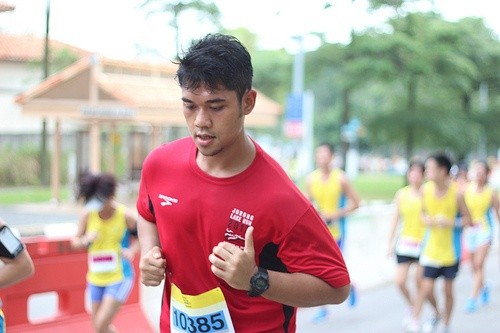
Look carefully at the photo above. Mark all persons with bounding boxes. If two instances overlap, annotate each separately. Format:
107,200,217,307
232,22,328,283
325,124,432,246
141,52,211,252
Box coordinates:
135,33,360,333
0,217,35,333
69,168,137,333
383,153,500,333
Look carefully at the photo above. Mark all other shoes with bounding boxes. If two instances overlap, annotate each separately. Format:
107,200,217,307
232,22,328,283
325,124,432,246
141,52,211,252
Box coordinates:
403,313,422,333
462,296,478,314
310,307,327,323
424,316,440,333
480,285,490,306
442,325,449,333
349,284,356,307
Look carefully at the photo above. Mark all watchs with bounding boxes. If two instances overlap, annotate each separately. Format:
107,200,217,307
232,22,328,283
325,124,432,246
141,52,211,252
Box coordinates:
246,265,270,297
